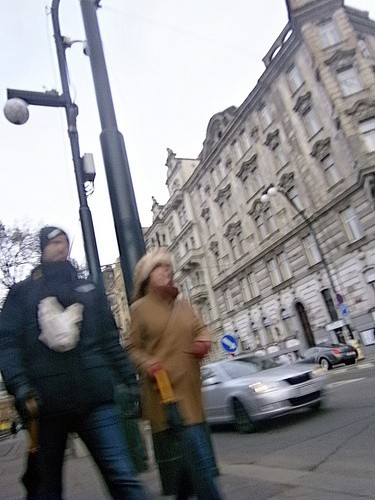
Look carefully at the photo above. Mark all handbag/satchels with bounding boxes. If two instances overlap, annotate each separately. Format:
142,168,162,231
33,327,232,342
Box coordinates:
36,295,84,353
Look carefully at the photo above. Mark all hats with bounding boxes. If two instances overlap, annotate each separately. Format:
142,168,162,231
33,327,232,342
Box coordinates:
132,248,173,300
38,226,69,252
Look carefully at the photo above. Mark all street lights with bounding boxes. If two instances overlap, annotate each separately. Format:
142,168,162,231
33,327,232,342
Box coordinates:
3,1,105,301
259,187,356,341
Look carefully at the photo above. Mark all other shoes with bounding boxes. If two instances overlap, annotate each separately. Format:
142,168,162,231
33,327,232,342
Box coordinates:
175,480,221,500
141,492,162,500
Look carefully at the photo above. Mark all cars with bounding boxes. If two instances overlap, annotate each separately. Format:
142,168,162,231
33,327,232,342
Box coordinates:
295,343,359,371
201,353,323,433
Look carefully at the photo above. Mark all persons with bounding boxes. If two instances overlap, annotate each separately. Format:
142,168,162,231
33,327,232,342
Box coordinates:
0,225,156,500
124,250,223,500
